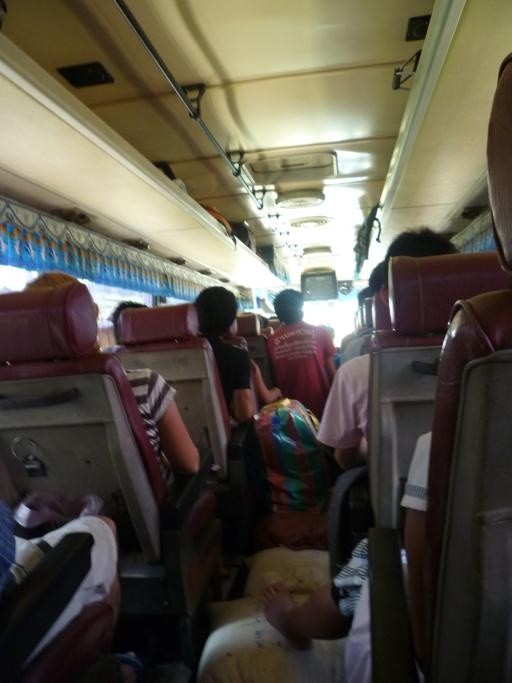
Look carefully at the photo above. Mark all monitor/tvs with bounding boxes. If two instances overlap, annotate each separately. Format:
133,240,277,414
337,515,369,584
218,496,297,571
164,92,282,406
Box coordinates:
302,271,338,300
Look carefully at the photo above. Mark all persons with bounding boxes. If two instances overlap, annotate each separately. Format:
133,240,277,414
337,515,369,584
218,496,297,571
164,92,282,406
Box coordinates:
262,431,463,683
316,225,461,470
266,289,335,420
112,287,284,422
21,273,201,478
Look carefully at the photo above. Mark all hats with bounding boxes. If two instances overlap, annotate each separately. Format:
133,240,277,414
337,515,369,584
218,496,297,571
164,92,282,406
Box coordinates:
13,494,104,554
195,287,237,336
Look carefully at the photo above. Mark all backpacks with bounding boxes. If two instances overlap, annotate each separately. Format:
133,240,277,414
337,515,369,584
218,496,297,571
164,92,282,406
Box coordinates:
203,204,256,252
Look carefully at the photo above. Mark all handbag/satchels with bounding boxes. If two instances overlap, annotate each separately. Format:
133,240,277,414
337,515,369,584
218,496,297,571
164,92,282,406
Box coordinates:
251,397,336,551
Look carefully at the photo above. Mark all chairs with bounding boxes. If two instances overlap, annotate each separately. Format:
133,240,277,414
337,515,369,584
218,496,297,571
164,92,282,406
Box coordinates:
2,283,226,638
2,563,125,683
235,313,281,391
114,303,256,553
339,253,511,682
